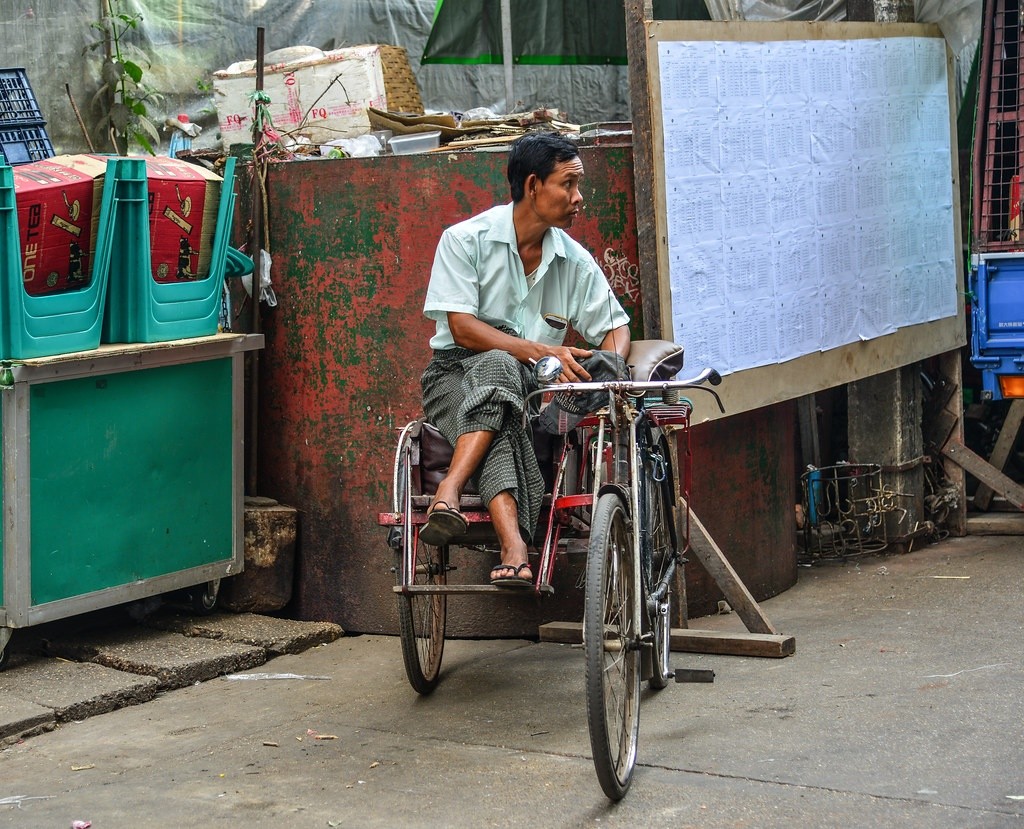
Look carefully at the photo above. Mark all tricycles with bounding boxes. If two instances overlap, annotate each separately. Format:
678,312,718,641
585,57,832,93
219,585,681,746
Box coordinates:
376,339,725,802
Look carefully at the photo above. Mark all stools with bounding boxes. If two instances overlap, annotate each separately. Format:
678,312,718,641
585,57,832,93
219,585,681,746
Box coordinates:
0,156,237,360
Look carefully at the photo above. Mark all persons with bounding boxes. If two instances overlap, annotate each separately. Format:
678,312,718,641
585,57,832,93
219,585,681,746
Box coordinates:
419,132,634,593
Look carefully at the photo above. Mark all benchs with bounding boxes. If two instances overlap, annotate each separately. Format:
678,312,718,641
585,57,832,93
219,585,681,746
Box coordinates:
408,340,684,509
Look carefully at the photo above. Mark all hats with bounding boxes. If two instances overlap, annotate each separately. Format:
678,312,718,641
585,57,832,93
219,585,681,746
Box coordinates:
539,348,630,435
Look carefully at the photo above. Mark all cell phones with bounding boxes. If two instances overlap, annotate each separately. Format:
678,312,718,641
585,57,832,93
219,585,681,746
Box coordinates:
543,313,568,329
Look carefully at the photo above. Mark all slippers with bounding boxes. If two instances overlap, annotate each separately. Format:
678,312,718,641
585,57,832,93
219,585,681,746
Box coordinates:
491,561,532,587
419,501,469,546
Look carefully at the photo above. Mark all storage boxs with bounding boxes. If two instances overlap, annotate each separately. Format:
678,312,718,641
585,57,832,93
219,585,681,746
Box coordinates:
0,125,56,166
0,67,47,126
213,47,386,155
11,156,109,298
89,150,225,283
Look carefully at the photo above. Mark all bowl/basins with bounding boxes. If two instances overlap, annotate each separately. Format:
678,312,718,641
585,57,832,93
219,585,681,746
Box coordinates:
388,131,442,154
